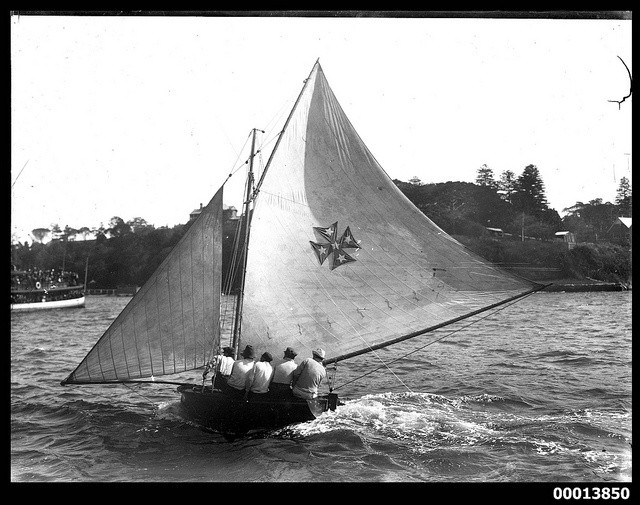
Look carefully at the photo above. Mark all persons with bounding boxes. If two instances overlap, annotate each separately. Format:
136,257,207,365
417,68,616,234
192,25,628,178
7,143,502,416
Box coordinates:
291,347,327,400
266,346,299,399
223,344,257,396
202,346,235,388
242,350,275,402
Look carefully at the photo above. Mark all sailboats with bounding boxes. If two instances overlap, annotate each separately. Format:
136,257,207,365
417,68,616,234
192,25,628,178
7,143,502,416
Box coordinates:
60,56,554,438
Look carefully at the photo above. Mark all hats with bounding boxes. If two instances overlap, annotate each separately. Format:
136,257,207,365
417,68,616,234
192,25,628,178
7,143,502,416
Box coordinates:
312,348,326,358
242,345,257,358
223,347,235,356
283,347,298,355
262,352,274,361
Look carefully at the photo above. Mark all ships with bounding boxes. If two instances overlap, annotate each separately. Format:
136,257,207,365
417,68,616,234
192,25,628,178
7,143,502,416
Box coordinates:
11,255,89,313
